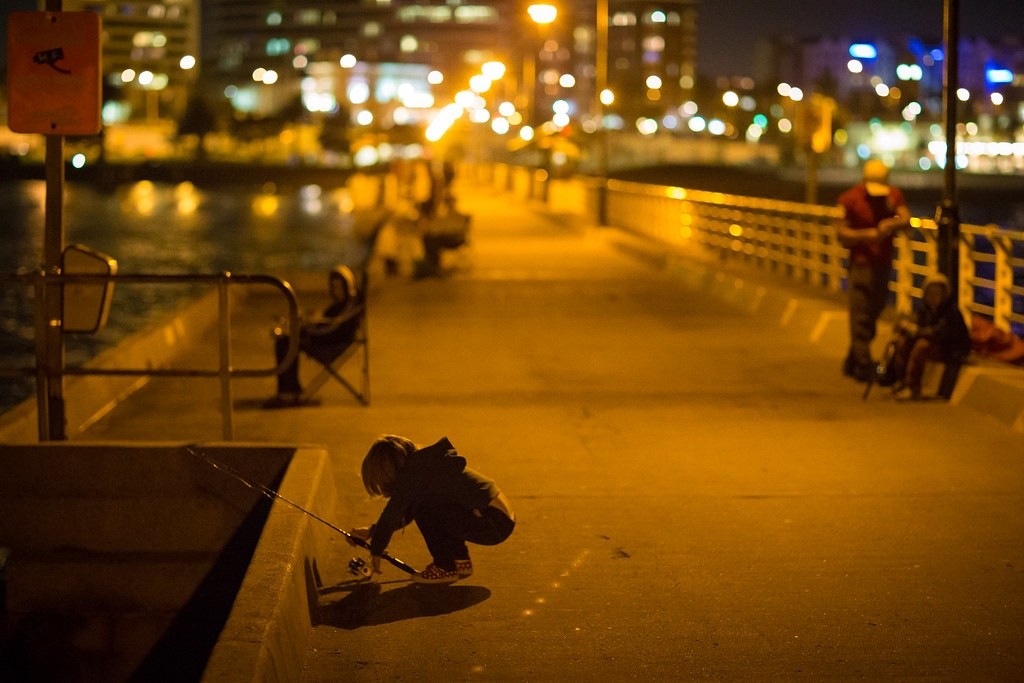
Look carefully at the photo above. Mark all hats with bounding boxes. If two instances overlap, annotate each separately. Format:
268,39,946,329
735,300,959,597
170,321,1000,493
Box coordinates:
865,159,891,196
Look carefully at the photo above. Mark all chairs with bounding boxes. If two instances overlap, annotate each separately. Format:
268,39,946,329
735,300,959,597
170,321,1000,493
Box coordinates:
291,270,370,407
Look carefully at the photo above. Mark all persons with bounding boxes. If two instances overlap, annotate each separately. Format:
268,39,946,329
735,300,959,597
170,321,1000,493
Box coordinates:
346,434,516,583
261,264,363,410
832,158,913,382
879,272,971,400
420,196,469,276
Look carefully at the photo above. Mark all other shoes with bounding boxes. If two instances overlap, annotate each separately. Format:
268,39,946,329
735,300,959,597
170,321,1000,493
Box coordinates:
842,357,881,383
894,384,920,402
878,370,903,387
264,395,300,408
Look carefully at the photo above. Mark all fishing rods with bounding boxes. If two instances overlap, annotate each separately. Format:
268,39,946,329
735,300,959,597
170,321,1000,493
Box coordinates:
860,288,915,402
186,446,420,574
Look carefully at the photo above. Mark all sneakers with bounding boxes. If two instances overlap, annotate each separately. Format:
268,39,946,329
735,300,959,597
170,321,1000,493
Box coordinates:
455,559,473,577
410,562,458,584
